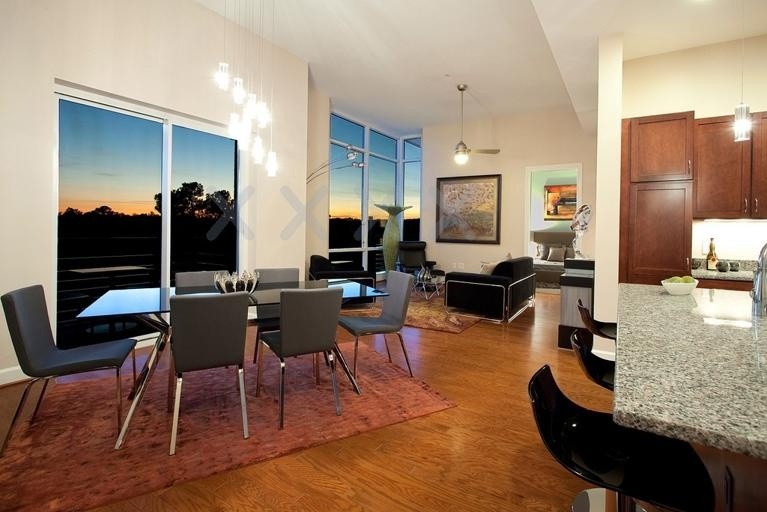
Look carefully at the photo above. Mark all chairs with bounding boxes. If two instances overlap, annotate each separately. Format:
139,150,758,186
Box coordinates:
309,255,377,312
398,241,445,300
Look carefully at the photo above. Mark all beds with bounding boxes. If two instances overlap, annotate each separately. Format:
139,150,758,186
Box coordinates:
530,231,576,289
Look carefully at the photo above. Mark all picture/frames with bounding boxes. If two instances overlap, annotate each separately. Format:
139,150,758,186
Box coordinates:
543,183,576,221
435,174,502,245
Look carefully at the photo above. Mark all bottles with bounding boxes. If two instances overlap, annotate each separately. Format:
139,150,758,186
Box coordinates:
706,237,719,270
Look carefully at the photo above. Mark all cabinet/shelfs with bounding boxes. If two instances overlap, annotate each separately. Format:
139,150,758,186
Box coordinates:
619,112,767,284
558,257,595,350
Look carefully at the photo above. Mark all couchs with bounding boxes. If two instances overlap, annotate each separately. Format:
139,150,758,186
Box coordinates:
444,256,536,325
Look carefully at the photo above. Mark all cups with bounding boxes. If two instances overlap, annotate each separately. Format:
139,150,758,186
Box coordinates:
691,258,739,272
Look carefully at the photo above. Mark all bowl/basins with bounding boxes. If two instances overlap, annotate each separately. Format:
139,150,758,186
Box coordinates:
660,278,700,295
211,269,262,298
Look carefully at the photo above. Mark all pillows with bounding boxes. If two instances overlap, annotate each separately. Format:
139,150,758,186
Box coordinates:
531,240,575,261
479,253,513,277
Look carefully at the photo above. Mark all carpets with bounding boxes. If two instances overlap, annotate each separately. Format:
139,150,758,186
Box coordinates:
339,284,486,333
1,340,459,512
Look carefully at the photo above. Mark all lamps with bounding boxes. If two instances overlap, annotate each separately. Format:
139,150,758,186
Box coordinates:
306,143,365,184
734,0,752,142
213,0,280,177
453,83,471,165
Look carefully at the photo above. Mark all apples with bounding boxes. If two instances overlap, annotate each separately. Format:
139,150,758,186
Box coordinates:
670,276,695,283
